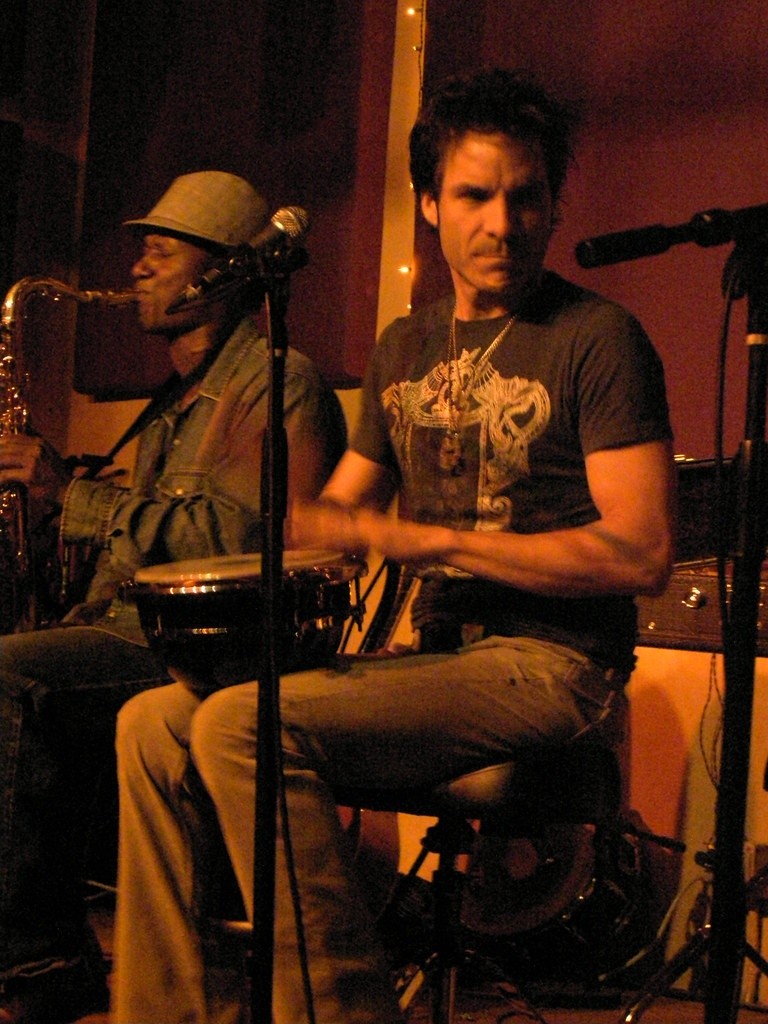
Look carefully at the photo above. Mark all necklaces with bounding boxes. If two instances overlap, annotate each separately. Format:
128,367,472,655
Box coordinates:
438,265,547,470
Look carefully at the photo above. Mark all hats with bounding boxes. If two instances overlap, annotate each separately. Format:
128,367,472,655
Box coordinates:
120,170,268,249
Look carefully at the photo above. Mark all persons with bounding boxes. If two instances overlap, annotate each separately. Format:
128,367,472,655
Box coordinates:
0,167,350,1024
108,85,676,1024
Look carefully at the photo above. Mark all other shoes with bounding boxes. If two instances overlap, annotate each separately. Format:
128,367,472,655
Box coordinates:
0,971,110,1024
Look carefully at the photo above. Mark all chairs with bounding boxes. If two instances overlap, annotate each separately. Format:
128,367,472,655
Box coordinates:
69,373,621,1022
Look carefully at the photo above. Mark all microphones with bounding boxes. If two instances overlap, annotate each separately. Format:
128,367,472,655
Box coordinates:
163,208,310,315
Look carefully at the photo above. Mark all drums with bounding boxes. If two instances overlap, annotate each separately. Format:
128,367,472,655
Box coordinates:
124,547,357,706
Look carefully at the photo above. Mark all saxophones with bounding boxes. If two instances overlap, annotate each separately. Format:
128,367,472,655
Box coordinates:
1,271,140,635
